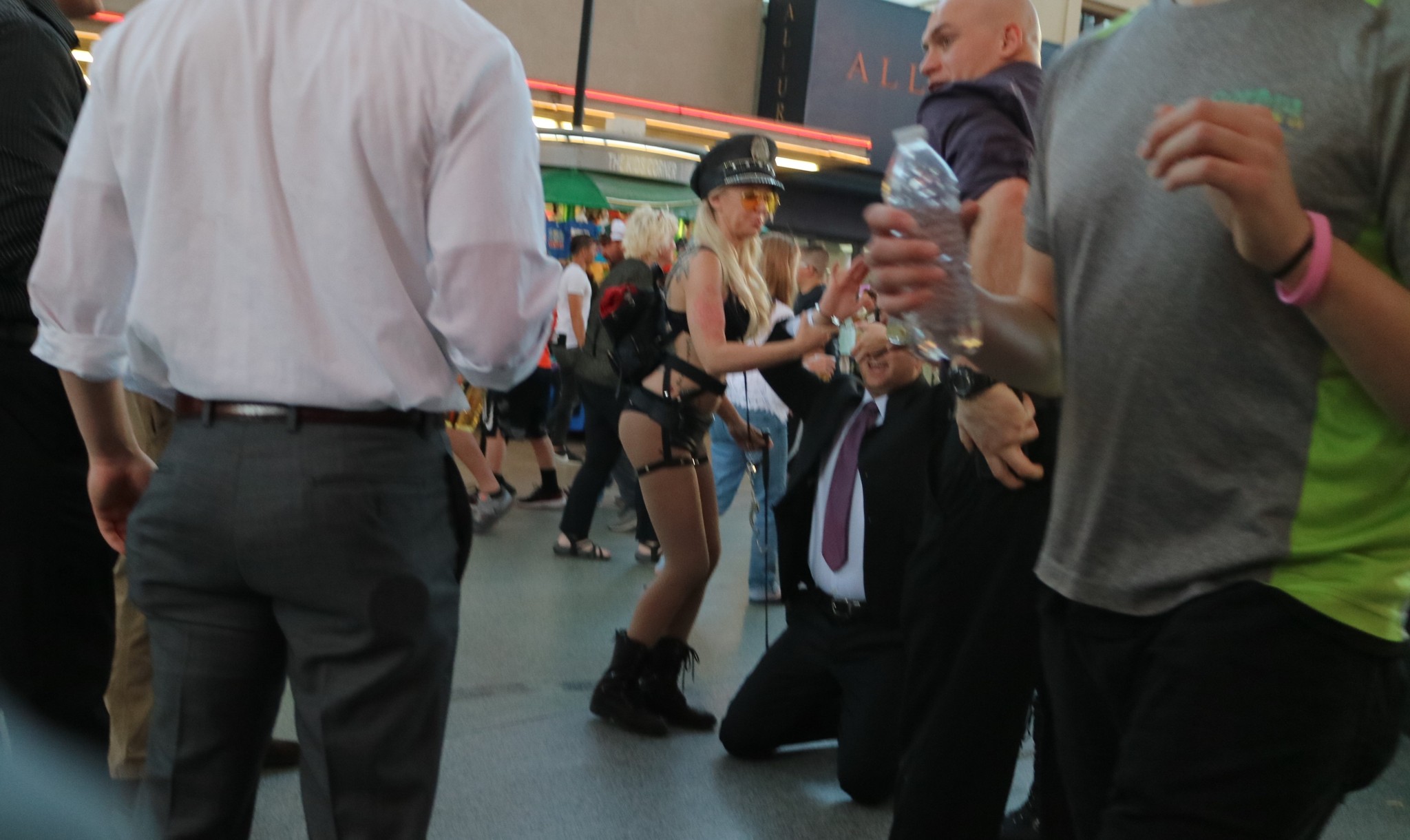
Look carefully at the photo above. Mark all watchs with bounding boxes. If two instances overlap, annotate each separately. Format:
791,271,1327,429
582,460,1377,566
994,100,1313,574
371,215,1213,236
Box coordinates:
945,362,995,402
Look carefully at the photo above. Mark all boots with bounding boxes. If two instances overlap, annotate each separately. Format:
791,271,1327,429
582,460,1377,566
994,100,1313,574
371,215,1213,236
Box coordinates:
651,638,717,731
590,629,670,737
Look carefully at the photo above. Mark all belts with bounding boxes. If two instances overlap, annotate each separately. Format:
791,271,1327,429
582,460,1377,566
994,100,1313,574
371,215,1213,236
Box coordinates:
178,394,445,429
813,595,865,621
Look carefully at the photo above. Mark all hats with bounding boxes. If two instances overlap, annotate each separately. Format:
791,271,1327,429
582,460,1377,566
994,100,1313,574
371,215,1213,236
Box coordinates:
690,133,785,201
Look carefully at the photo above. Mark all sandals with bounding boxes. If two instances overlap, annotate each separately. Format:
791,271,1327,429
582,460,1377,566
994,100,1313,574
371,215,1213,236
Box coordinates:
635,540,661,562
554,532,611,561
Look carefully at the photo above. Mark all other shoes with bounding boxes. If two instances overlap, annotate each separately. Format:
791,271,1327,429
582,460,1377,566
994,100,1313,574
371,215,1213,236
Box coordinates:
554,447,584,465
472,485,514,533
263,739,301,773
518,486,565,508
607,495,637,532
749,587,789,603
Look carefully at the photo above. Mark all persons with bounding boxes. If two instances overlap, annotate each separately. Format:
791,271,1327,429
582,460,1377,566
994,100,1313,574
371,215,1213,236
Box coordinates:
587,133,870,738
25,1,557,840
863,1,1410,840
0,1,1410,840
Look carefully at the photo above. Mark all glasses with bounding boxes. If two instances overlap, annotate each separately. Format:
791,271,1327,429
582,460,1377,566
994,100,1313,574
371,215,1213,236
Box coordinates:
742,189,781,214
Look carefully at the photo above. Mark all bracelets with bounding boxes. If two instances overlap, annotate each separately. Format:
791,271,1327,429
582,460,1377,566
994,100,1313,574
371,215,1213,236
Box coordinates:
1271,210,1333,309
813,301,847,328
1255,232,1315,285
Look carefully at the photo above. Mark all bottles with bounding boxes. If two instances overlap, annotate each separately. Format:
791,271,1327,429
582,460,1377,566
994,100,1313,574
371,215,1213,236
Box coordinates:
886,125,982,361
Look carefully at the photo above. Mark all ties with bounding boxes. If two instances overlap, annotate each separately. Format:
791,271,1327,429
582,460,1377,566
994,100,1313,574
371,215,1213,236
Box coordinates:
821,401,881,572
